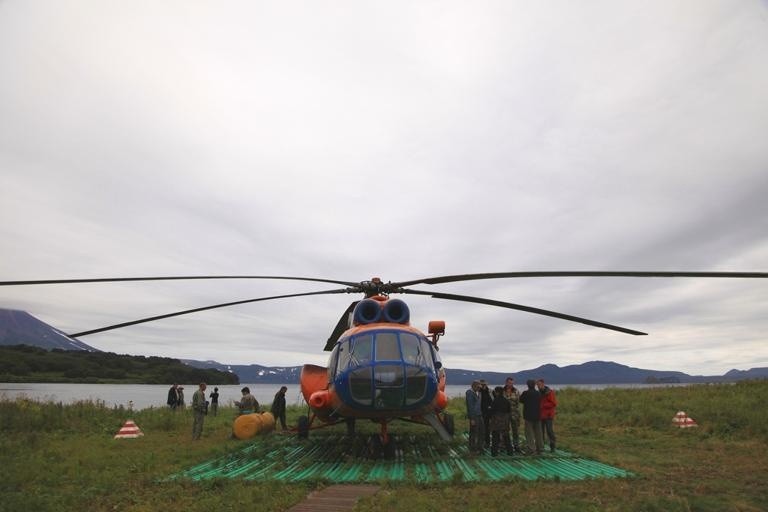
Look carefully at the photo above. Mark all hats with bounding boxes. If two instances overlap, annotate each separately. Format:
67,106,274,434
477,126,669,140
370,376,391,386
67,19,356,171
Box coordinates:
178,385,184,390
480,379,489,385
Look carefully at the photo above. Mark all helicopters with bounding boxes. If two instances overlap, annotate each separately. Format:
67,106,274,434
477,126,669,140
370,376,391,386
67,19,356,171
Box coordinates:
0,270,768,460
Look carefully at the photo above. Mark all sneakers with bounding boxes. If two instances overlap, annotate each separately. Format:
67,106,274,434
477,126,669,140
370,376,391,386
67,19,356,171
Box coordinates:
515,445,556,455
490,447,513,457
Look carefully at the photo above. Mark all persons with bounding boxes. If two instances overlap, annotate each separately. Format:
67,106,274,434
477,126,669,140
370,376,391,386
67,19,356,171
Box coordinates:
270,386,290,432
466,377,558,457
227,387,259,440
167,383,180,409
205,399,209,415
210,387,219,417
191,383,206,441
178,386,184,406
